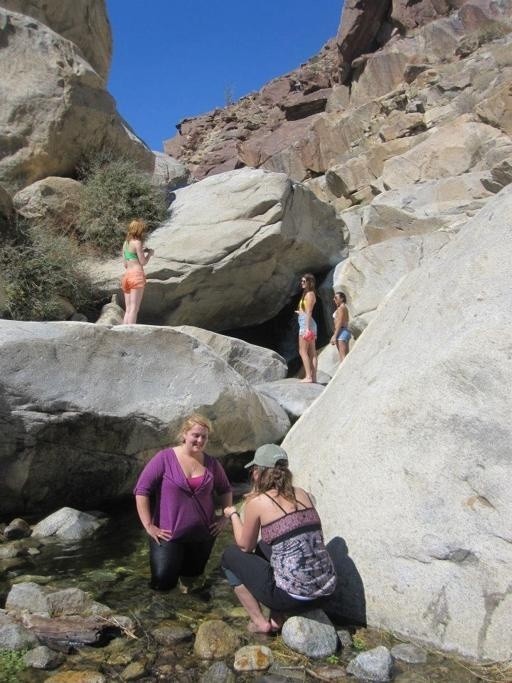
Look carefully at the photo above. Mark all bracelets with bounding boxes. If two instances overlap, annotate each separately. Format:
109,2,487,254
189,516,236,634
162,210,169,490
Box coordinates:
229,511,240,520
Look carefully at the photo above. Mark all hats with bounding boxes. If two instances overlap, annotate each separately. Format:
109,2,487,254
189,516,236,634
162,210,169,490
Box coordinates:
245,444,289,467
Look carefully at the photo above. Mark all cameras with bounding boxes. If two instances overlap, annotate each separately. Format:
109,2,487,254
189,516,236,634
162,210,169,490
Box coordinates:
144,248,150,252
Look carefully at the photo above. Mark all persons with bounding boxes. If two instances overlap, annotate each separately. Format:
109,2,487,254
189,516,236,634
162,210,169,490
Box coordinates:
293,272,320,384
330,292,351,361
133,413,235,592
122,219,156,325
220,441,337,632
